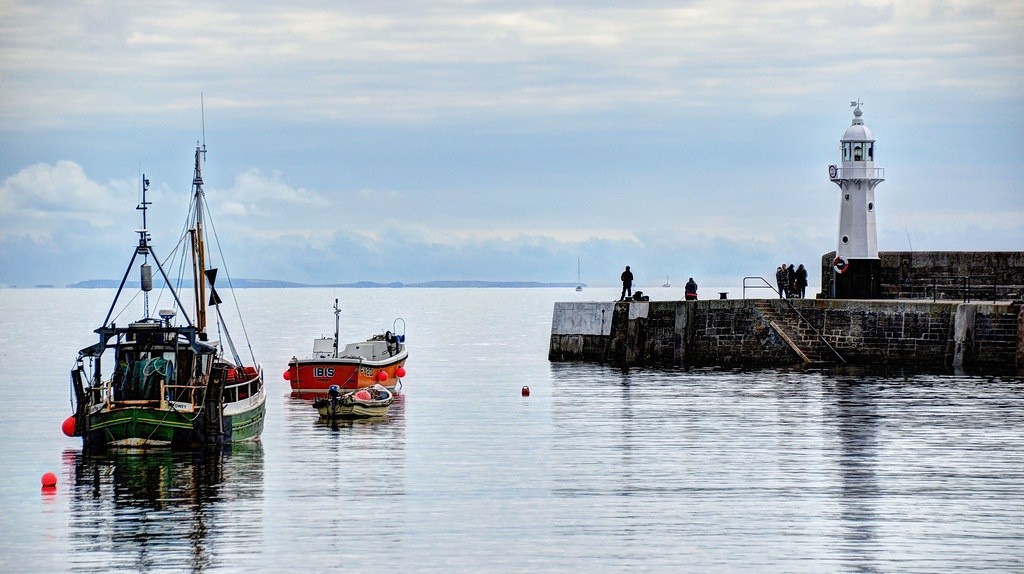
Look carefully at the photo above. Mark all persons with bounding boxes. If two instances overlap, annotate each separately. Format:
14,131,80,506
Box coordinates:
776,263,795,298
619,266,633,301
795,264,807,299
685,278,697,300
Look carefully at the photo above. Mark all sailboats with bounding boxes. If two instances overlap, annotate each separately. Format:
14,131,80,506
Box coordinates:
62,91,268,448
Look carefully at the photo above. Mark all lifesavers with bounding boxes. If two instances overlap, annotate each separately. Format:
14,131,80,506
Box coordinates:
833,256,848,273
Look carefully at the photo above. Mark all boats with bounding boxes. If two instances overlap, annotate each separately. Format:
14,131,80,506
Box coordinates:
282,298,409,392
62,440,270,574
311,383,393,416
